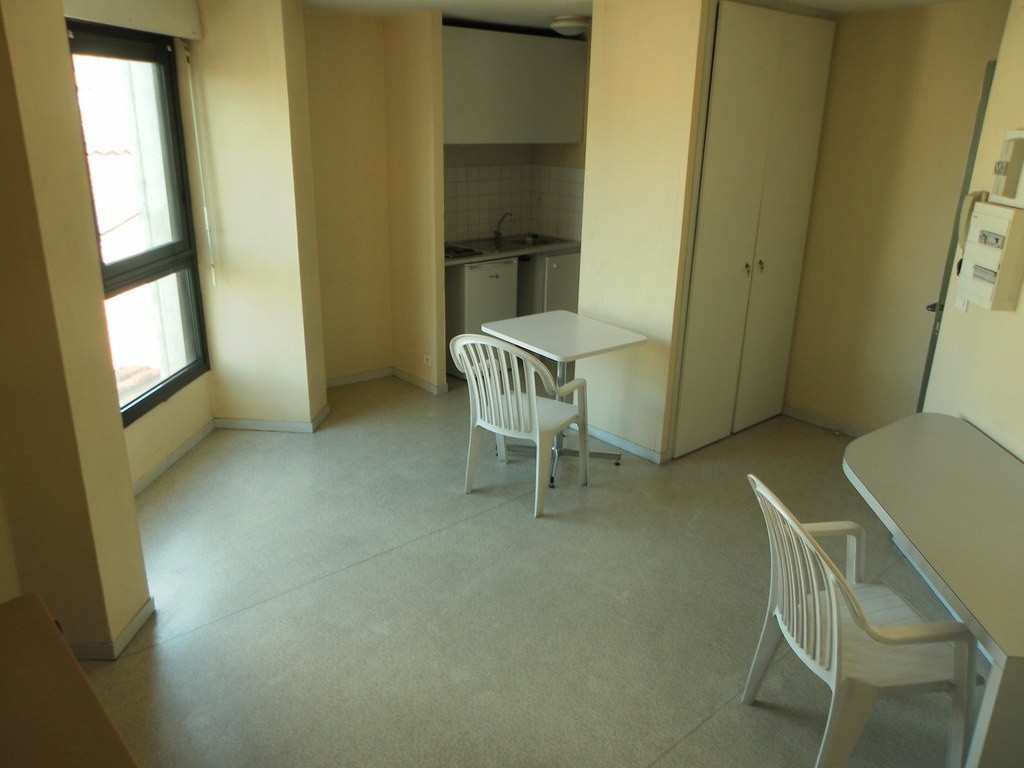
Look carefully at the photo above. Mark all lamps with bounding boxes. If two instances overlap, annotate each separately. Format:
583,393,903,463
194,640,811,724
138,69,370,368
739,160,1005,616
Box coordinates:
549,15,590,35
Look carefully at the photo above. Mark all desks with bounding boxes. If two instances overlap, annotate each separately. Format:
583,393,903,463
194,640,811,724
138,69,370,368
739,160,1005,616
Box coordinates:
480,309,647,489
841,412,1024,768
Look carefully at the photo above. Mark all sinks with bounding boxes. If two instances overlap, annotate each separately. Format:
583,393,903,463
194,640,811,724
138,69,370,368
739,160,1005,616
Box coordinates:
509,236,561,249
464,240,522,254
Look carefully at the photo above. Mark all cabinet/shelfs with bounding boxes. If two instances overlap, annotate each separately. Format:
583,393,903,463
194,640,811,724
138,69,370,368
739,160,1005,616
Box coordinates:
569,1,839,464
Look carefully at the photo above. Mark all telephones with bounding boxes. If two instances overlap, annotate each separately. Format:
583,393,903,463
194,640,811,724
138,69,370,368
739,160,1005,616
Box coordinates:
958,191,990,249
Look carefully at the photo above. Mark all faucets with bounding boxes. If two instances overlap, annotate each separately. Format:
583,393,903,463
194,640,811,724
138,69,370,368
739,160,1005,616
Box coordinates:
494,212,516,243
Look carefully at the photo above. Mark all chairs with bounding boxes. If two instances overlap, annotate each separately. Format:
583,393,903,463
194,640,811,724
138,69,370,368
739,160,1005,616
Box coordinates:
741,474,977,768
449,333,591,518
